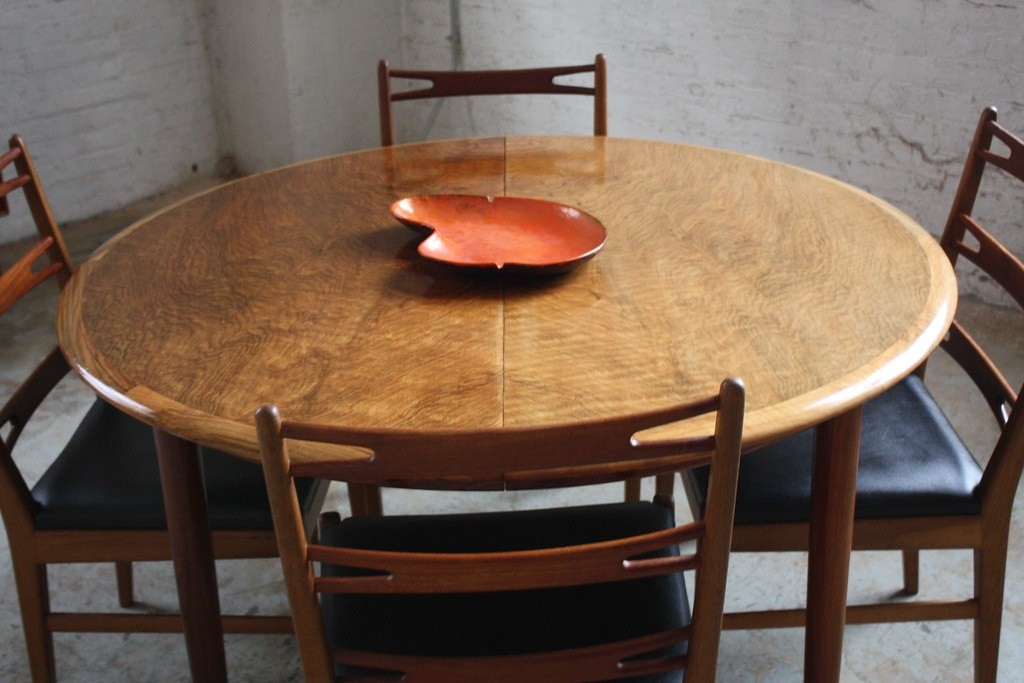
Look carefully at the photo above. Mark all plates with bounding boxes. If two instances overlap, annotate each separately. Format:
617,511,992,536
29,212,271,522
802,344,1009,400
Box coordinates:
390,193,608,270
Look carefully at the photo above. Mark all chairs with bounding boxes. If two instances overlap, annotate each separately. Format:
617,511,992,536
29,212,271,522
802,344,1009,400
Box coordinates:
649,108,1023,683
250,373,747,683
376,51,609,157
0,131,339,683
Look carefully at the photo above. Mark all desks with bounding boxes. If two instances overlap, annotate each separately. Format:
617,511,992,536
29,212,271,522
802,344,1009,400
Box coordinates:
57,125,956,679
